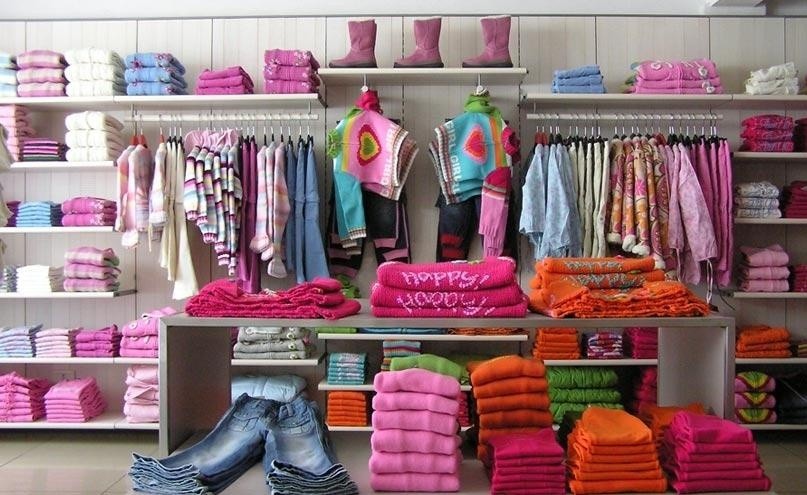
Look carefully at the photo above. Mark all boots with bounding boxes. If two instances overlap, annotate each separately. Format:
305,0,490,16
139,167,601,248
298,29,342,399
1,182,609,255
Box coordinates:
395,16,444,68
328,18,379,70
463,16,514,69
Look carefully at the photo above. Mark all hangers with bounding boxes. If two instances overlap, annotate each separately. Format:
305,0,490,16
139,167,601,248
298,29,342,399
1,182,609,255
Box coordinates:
531,110,721,140
130,112,314,146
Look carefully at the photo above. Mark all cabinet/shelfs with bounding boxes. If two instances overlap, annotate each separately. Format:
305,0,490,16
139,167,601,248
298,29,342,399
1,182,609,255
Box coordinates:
521,90,806,434
0,90,331,432
313,62,532,441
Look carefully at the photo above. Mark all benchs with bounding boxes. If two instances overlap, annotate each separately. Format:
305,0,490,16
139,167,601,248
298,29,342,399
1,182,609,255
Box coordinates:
155,314,735,460
